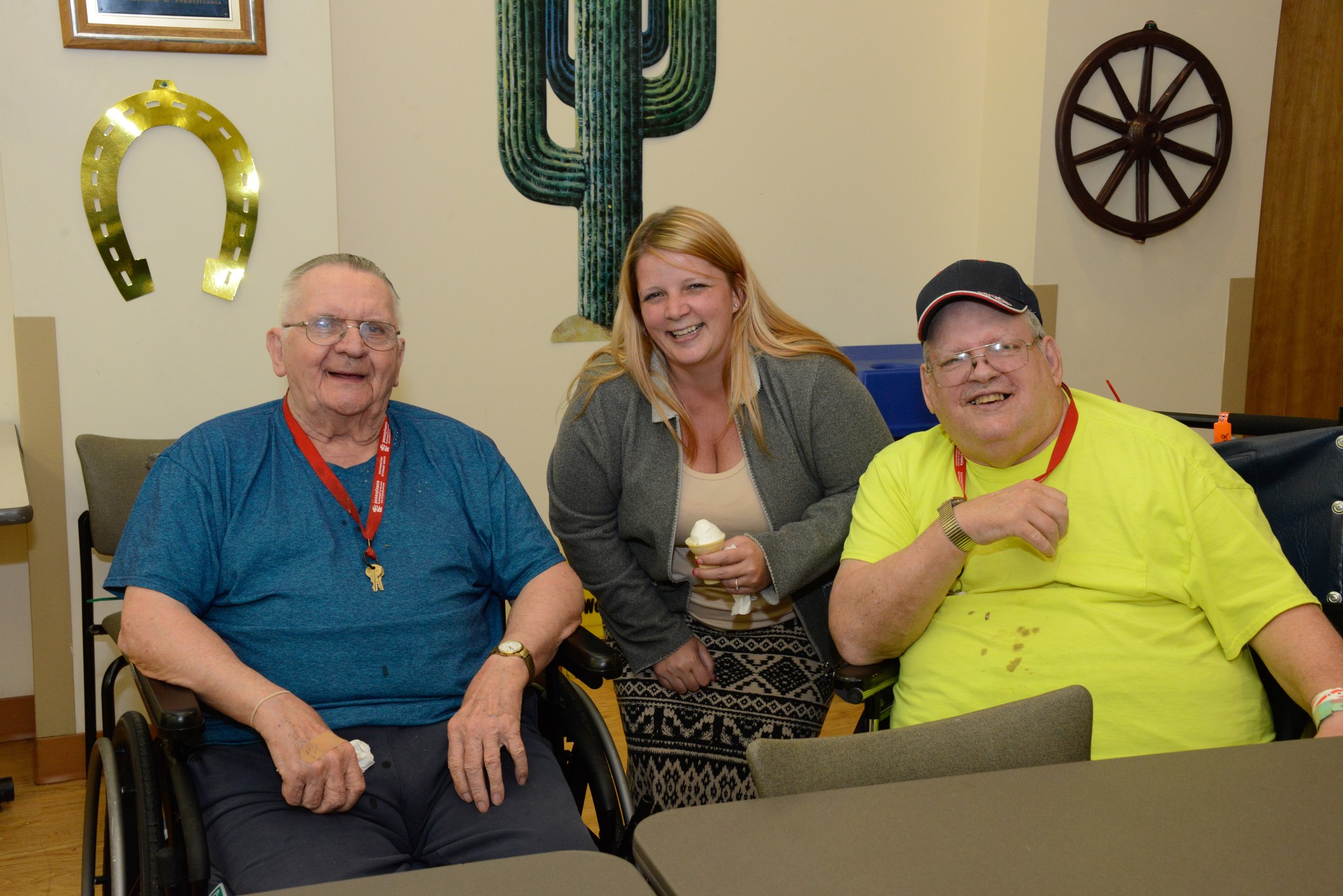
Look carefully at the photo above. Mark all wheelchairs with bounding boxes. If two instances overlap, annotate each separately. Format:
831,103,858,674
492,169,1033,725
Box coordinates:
71,433,650,895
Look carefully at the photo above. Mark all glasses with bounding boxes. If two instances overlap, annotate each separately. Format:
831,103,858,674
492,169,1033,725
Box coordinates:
282,316,401,352
925,336,1044,388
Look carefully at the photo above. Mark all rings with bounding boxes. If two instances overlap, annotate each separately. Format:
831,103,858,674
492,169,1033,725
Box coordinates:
735,579,738,591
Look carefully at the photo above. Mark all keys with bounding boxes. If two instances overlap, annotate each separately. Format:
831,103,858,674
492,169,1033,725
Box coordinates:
365,564,381,592
377,566,384,591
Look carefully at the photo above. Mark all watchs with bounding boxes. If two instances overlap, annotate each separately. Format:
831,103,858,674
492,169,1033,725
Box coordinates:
937,497,976,553
487,640,535,684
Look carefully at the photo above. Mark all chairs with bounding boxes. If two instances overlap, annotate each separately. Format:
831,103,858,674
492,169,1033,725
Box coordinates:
745,685,1094,797
75,434,179,778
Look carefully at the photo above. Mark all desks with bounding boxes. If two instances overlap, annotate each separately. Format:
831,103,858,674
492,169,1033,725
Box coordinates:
632,735,1342,896
247,850,653,896
0,422,34,526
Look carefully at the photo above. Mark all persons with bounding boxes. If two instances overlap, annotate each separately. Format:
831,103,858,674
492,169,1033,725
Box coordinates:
548,208,895,814
103,254,598,896
828,260,1343,743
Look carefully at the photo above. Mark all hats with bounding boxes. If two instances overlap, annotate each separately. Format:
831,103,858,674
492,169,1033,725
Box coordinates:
916,259,1043,348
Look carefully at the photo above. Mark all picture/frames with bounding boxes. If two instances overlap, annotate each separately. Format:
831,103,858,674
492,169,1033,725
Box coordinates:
58,0,267,55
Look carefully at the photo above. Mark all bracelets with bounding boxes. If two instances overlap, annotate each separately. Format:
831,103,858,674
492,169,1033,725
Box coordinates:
250,691,292,729
1311,687,1343,714
1313,700,1343,733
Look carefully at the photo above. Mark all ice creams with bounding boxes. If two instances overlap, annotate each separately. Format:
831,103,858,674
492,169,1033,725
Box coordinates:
685,519,725,585
